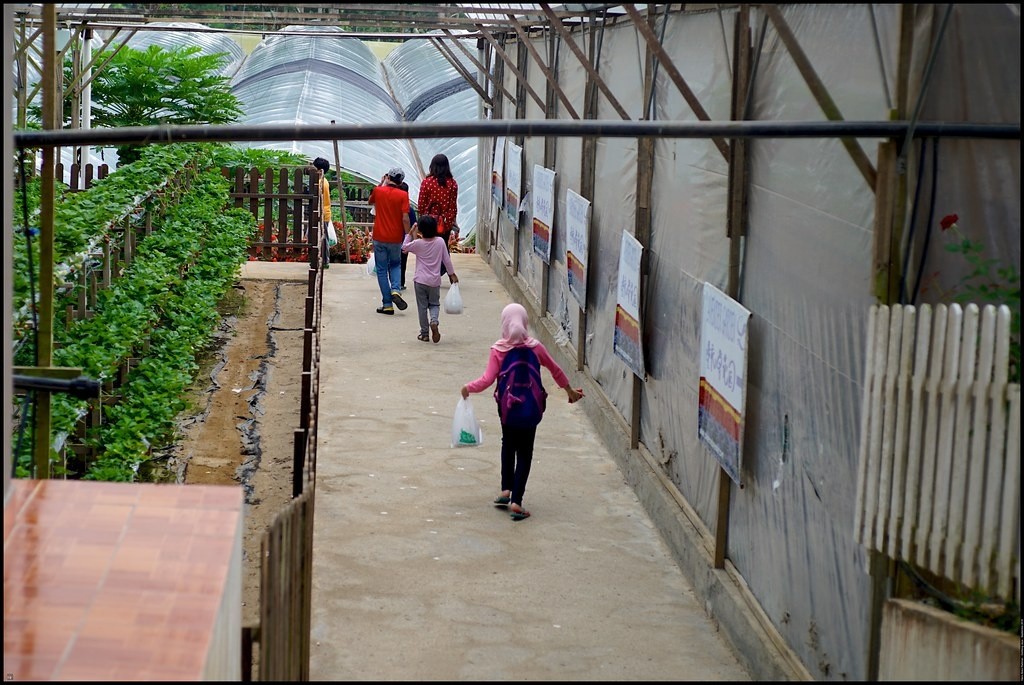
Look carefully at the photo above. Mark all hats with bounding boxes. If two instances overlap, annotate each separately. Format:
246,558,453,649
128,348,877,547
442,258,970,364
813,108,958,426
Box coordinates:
384,167,405,181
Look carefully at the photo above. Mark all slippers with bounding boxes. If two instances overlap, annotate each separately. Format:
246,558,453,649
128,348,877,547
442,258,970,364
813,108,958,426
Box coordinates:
510,507,530,518
493,495,510,504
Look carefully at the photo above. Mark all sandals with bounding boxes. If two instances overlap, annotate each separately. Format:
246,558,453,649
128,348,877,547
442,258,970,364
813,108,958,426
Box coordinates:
418,334,429,342
431,323,440,343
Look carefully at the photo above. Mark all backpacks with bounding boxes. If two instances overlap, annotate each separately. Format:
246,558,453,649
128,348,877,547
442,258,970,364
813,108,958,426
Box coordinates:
494,347,548,427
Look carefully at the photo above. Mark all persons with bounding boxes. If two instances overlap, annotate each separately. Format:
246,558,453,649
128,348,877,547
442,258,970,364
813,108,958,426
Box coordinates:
308,157,338,269
401,216,464,343
450,303,585,518
389,182,417,289
419,153,459,277
368,167,409,315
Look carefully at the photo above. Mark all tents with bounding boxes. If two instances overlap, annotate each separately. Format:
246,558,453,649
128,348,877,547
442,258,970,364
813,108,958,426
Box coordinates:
16,18,481,240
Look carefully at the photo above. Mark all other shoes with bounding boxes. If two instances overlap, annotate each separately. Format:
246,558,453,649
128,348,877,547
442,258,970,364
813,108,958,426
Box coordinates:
391,292,408,310
376,306,394,315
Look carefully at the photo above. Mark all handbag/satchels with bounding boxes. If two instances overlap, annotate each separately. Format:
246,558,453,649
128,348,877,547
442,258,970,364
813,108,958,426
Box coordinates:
443,282,463,314
423,202,450,234
327,219,337,247
450,394,483,449
